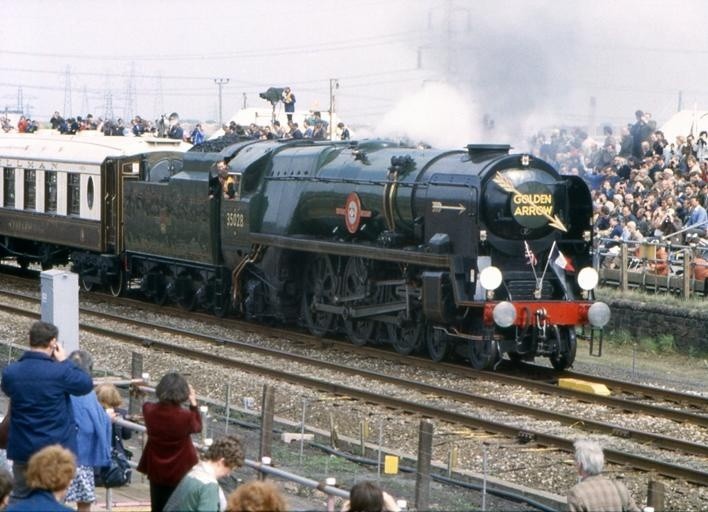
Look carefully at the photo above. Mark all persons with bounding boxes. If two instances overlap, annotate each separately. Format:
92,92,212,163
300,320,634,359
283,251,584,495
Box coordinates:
217,170,238,199
17,111,183,138
226,479,285,511
567,440,640,512
222,111,328,142
163,435,245,511
2,323,123,510
282,87,295,124
337,122,349,141
326,478,398,511
207,160,227,200
531,111,708,280
191,124,205,146
137,373,203,511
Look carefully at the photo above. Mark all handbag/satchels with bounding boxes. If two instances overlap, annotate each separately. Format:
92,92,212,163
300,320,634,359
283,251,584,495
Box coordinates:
101,455,131,487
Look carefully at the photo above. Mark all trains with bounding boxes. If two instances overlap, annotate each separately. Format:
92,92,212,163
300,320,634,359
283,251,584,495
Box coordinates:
0,129,611,369
